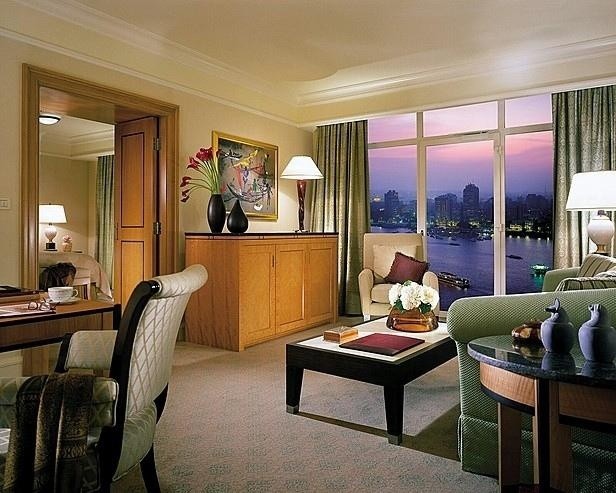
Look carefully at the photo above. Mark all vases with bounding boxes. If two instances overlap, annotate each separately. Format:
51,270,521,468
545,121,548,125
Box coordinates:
62,242,72,252
386,309,439,332
207,194,226,233
227,200,248,233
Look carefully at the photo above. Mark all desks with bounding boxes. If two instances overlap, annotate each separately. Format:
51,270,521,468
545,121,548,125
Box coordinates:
0,300,121,355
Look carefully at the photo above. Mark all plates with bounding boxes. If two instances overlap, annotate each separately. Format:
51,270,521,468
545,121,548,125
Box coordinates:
45,297,82,304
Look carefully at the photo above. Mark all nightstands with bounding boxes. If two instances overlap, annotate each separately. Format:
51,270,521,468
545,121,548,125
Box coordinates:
273,232,339,339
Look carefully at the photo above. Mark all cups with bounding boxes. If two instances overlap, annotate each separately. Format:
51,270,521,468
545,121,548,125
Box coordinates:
47,286,78,302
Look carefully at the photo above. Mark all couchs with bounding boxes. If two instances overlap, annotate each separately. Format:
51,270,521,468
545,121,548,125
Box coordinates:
447,267,616,493
358,233,439,322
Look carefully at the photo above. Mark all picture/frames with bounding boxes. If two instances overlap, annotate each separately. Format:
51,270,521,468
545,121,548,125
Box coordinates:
211,130,279,221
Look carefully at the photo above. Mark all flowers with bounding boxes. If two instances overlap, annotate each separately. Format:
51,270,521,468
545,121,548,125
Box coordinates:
388,280,440,315
63,233,72,242
180,144,222,203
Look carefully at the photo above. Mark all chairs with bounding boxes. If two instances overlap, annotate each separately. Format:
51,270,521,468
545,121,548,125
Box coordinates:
72,269,90,301
0,264,208,493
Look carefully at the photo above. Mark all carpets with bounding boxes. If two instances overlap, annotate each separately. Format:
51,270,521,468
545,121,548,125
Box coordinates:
109,319,498,493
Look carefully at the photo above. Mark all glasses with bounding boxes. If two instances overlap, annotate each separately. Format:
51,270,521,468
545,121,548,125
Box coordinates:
28,301,59,311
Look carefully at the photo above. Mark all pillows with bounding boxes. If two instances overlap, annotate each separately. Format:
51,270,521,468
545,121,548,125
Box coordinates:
384,252,431,286
555,278,616,291
577,254,616,277
373,244,416,284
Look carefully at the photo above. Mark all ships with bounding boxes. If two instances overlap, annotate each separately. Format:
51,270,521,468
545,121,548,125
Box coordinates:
437,271,471,289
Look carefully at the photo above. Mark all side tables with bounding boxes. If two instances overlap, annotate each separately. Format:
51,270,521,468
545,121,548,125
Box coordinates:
469,336,616,493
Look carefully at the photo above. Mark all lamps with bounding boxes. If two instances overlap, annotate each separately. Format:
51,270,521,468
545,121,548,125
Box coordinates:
566,171,616,256
39,203,67,251
280,155,324,232
38,114,61,126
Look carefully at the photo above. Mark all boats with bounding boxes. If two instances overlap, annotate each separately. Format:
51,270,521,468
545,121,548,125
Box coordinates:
532,264,550,274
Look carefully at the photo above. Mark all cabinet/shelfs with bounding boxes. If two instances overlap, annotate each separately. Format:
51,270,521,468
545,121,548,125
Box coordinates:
39,252,112,301
184,232,274,352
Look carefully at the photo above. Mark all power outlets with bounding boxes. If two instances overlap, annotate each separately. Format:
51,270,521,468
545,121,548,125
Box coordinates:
1,197,10,209
